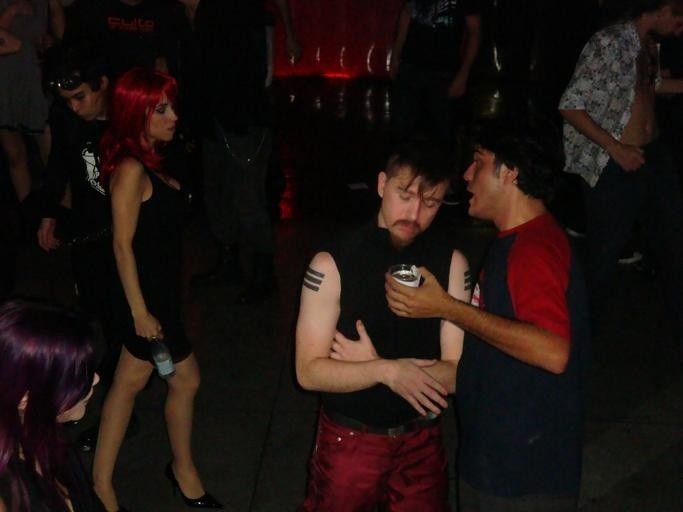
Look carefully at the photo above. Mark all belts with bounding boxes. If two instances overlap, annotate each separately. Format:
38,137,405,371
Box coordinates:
323,408,442,438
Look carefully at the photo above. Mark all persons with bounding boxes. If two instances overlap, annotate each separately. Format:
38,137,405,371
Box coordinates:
1,1,65,202
0,295,109,512
45,2,195,94
384,1,484,207
293,137,474,511
556,1,682,334
92,66,223,512
172,0,304,305
385,125,592,512
30,51,136,452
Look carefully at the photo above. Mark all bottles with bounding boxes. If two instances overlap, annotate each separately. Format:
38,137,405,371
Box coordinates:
149,339,176,379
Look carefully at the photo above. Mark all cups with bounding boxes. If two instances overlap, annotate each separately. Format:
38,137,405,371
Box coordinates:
388,263,421,289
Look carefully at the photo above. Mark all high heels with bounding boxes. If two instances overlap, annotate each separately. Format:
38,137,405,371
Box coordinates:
165,459,216,511
